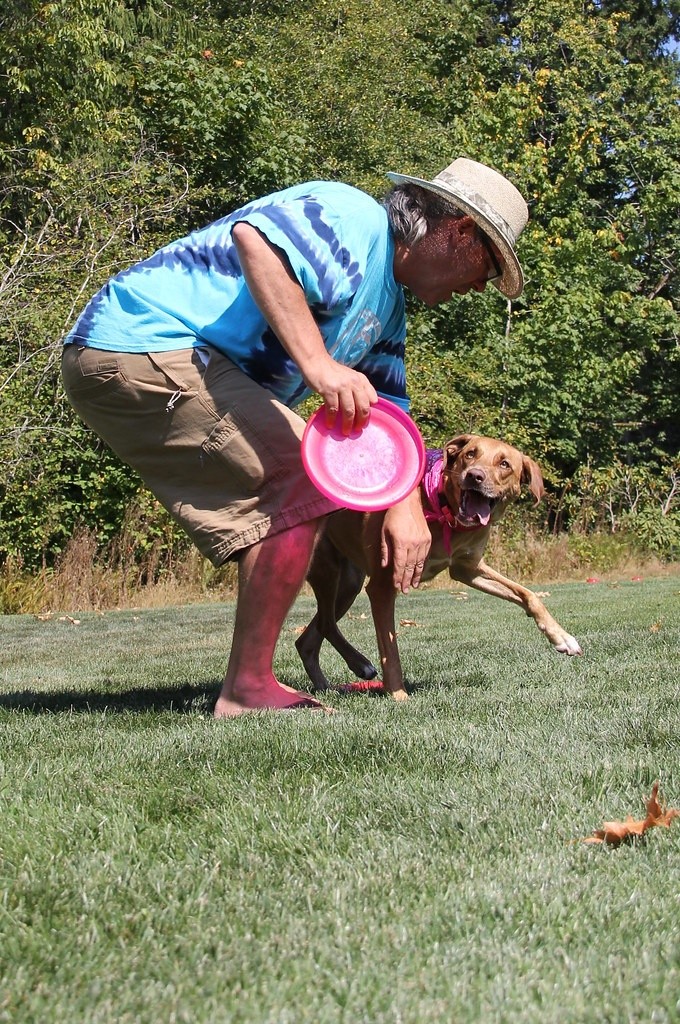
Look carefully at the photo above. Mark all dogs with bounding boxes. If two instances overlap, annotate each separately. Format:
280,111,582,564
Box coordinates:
294,432,583,702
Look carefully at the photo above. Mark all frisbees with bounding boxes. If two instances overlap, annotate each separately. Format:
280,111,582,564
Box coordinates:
296,394,426,512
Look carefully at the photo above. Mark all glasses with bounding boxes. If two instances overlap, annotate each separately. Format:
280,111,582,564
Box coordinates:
474,229,503,287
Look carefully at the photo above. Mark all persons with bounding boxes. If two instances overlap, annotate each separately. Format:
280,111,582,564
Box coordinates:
59,156,531,718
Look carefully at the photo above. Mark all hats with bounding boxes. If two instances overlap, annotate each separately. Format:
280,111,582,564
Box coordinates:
385,156,529,297
